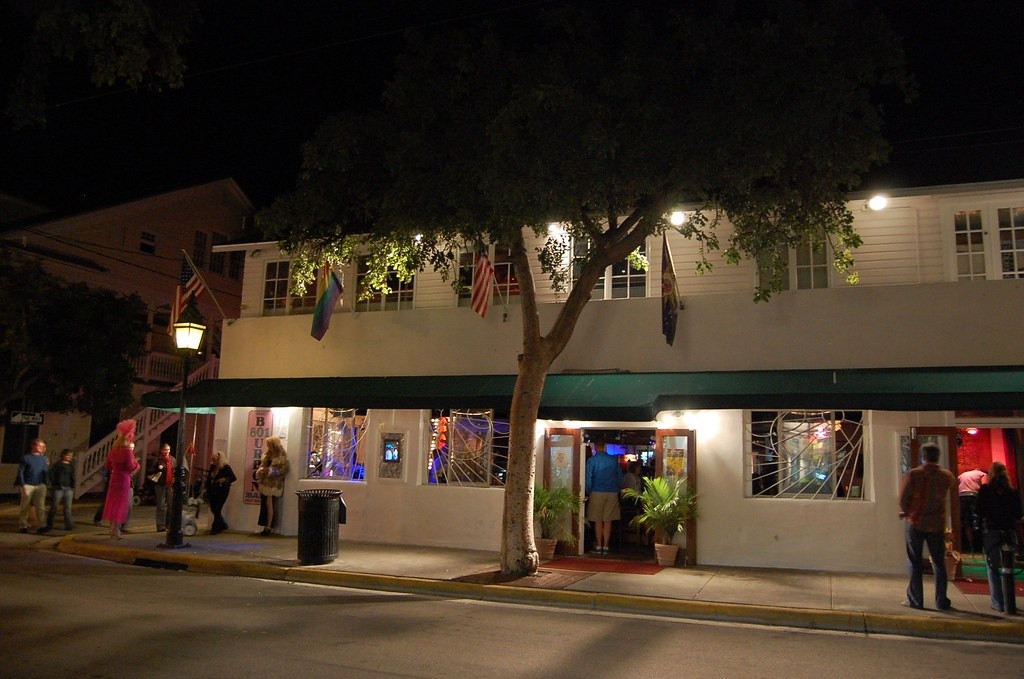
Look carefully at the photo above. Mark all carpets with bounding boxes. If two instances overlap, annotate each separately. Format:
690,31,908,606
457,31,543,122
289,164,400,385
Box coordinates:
951,575,1024,597
538,557,669,575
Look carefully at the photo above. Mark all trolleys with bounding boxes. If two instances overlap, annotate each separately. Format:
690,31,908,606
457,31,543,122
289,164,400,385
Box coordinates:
181,489,206,537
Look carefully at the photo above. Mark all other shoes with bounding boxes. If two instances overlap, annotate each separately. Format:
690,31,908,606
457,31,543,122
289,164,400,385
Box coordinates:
590,549,602,554
603,549,608,555
21,528,28,533
37,528,49,535
109,528,125,540
94,518,101,526
120,527,128,533
901,600,910,606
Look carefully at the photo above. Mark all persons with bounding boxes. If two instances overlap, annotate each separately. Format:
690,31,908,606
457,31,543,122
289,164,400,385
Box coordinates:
147,442,177,532
970,462,1021,616
957,466,990,555
254,436,289,537
201,450,237,536
898,443,954,611
92,418,140,542
44,449,78,533
585,437,655,557
309,453,322,477
19,438,51,535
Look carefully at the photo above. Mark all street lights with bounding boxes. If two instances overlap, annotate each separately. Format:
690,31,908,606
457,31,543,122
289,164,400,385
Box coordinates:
153,289,208,551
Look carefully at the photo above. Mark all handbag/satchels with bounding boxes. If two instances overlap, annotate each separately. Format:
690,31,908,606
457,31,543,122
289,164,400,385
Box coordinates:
148,472,163,483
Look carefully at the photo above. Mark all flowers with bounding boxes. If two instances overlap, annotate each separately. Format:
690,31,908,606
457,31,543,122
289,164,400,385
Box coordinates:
945,527,959,553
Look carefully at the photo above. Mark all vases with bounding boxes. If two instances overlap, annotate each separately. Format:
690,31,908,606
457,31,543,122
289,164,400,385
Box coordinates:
929,551,964,581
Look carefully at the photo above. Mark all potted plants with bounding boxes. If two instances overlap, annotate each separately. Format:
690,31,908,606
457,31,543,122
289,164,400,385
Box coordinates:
534,485,590,561
619,467,700,569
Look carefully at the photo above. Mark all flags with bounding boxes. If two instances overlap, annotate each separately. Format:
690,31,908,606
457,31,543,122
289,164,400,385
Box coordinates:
167,255,205,336
311,260,345,341
471,240,494,320
661,231,678,347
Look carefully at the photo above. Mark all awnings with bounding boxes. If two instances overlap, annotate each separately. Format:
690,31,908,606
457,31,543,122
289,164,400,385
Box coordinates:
140,362,1024,418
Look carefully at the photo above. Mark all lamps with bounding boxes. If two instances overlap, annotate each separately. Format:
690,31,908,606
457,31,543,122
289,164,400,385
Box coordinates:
249,249,262,258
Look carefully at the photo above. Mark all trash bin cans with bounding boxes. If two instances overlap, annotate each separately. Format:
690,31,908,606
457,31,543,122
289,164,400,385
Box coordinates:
294,488,343,565
